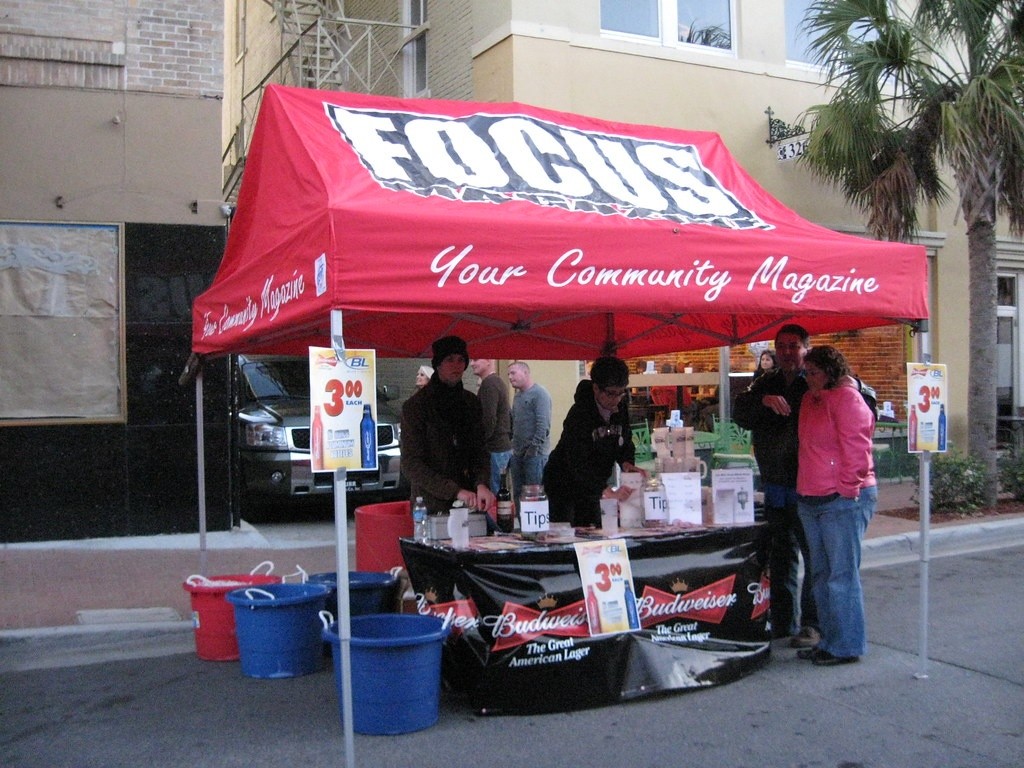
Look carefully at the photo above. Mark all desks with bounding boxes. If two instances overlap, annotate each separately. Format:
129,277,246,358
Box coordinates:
659,429,721,470
872,419,908,484
396,521,775,714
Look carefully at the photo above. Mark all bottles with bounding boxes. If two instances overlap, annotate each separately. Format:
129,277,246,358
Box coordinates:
640,477,670,527
411,497,429,542
519,485,550,540
497,474,512,532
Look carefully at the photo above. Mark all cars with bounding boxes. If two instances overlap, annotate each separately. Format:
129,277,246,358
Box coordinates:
232,353,402,522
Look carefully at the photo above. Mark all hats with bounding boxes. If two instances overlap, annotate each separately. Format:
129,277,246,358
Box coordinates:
431,335,469,372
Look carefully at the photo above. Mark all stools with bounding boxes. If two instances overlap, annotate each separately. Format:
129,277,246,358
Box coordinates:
872,444,894,483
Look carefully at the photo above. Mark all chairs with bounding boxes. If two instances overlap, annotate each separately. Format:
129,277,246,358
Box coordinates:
630,412,755,473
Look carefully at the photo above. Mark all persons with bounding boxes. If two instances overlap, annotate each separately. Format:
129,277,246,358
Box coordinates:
400,336,496,517
469,358,514,498
543,357,646,529
629,349,780,432
790,345,878,666
506,362,550,519
730,324,876,649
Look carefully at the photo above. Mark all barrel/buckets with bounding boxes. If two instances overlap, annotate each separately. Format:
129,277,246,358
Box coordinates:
319,607,452,735
183,561,280,660
227,573,330,678
297,565,403,660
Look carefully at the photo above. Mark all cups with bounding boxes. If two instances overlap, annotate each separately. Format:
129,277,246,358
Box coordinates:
599,498,618,532
450,508,469,549
683,367,693,374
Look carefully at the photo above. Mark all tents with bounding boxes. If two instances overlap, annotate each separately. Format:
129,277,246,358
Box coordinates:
191,85,932,768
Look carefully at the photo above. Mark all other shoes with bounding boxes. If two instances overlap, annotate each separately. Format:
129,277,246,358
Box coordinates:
789,626,822,648
795,646,824,660
765,628,799,639
812,652,861,667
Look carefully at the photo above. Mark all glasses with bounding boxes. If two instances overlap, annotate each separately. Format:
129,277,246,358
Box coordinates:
600,385,626,400
